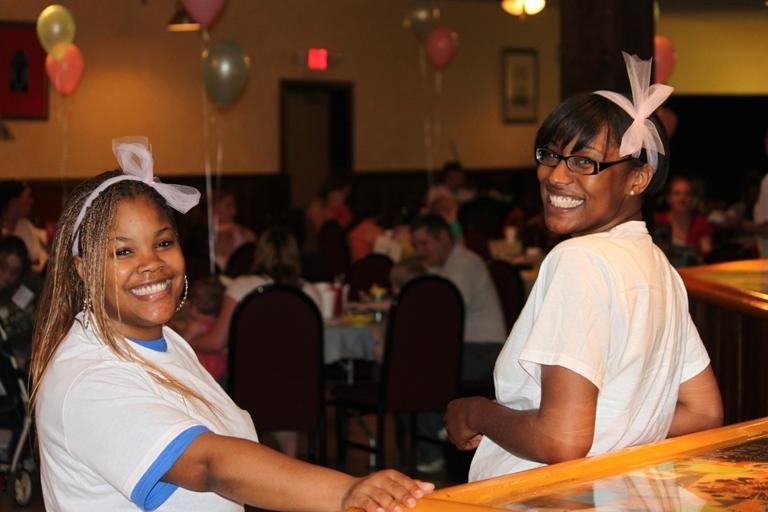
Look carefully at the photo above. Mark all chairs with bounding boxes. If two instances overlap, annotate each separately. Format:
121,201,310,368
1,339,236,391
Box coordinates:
330,273,467,475
227,283,326,466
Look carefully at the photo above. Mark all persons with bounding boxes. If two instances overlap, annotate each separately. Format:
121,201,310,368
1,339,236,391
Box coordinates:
27,135,434,512
184,271,227,381
303,173,354,255
408,214,508,358
0,182,48,272
653,177,714,267
422,162,475,240
708,134,768,257
0,236,42,369
445,51,724,485
189,228,324,458
204,188,257,271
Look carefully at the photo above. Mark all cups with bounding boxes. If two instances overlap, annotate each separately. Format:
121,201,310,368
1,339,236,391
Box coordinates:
314,283,330,298
321,291,336,320
331,287,342,318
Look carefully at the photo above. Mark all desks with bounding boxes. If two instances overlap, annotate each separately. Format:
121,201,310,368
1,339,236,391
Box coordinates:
372,413,768,511
676,259,768,422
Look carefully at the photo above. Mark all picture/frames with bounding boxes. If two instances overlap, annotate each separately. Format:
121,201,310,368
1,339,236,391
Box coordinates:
501,46,539,124
0,18,49,122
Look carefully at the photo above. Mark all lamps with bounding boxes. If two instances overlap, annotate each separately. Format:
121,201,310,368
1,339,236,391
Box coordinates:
166,1,201,32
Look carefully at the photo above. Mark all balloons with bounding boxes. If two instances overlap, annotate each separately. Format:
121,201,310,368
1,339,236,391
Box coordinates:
401,0,442,39
422,23,459,70
35,6,77,59
46,46,87,97
183,0,223,26
654,106,675,143
200,40,248,109
652,36,676,85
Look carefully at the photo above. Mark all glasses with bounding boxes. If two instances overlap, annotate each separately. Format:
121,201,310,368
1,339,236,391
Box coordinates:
535,146,634,176
